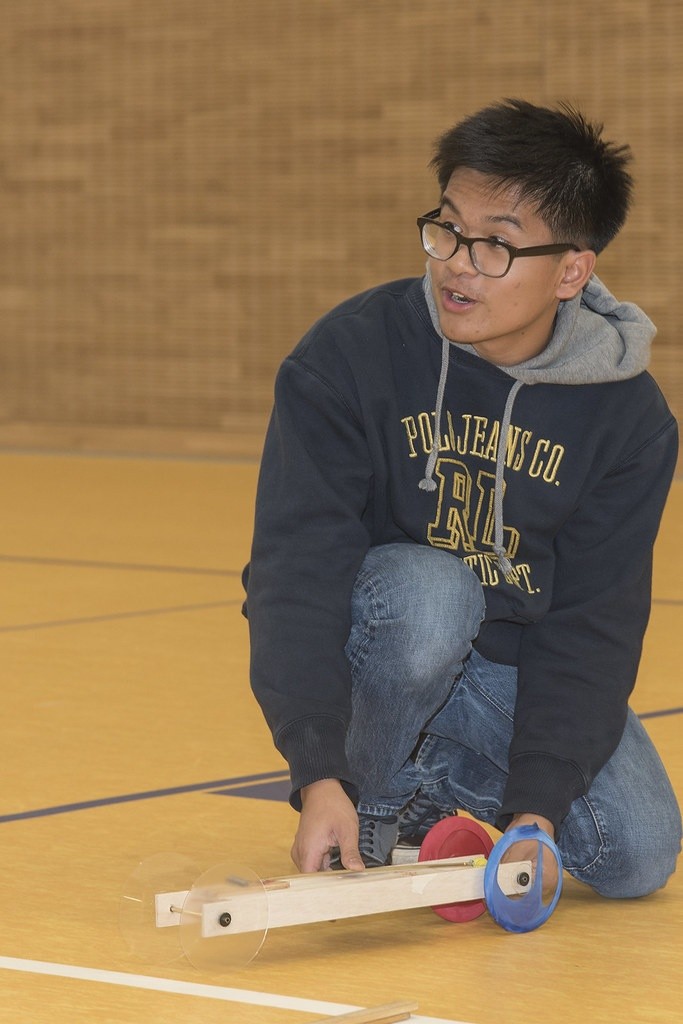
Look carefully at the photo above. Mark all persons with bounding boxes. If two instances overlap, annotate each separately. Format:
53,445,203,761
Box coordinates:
243,97,683,899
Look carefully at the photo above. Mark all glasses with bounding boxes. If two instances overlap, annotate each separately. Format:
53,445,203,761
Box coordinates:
416,207,582,279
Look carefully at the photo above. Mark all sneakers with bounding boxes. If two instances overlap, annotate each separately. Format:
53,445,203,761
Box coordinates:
396,793,458,847
327,814,399,870
